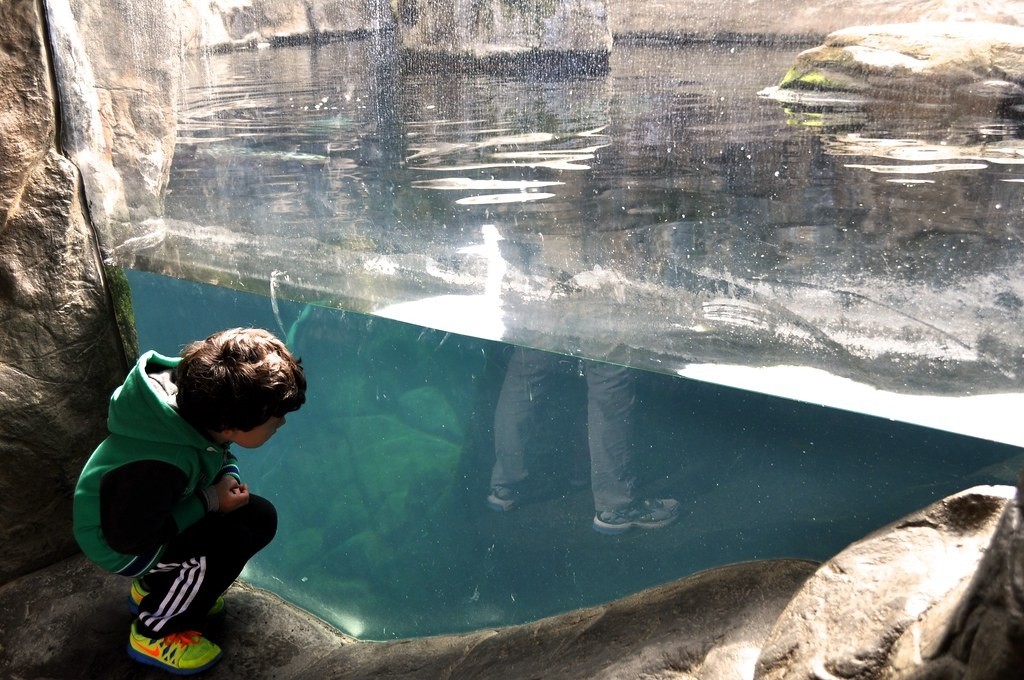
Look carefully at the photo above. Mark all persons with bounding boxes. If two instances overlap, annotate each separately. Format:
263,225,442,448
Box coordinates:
72,329,305,674
482,335,683,532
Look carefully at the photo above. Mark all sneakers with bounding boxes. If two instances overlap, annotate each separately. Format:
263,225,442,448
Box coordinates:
127,624,225,677
483,477,568,518
592,496,682,536
128,577,224,613
